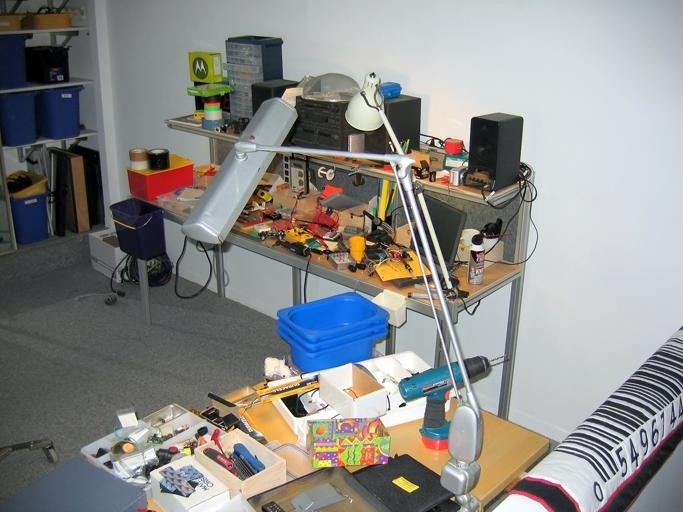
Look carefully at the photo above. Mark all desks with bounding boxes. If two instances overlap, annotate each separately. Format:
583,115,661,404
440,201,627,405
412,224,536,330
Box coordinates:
0,376,553,512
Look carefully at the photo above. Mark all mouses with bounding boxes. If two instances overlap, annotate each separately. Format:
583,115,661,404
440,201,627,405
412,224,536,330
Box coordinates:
440,276,459,289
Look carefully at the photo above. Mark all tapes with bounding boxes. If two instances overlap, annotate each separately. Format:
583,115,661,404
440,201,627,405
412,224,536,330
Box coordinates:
129,149,170,171
202,109,225,130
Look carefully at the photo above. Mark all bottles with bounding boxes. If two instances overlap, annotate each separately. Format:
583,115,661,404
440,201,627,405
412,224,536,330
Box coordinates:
111,440,135,456
466,236,486,284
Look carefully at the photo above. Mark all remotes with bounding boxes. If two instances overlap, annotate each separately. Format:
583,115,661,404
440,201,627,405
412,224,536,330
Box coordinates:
261,501,285,512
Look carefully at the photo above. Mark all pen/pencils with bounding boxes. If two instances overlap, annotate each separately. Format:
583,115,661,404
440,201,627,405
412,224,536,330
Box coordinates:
389,139,410,154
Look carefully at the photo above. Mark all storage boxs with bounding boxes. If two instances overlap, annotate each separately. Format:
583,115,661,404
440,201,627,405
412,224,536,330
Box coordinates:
88,228,131,283
127,154,193,203
276,291,389,373
0,14,84,244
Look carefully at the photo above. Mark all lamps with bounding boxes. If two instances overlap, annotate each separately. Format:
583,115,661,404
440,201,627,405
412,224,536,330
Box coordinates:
345,73,485,512
180,89,487,512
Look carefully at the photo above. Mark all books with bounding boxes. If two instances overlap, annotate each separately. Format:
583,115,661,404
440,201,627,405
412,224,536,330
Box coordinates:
168,111,230,127
176,188,203,201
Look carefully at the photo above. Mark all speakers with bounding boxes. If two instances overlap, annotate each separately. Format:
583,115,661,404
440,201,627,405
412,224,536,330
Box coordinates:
366,94,421,166
465,113,524,192
251,79,297,116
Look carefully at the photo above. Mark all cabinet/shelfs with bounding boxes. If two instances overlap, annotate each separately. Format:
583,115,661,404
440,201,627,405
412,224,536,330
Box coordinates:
0,0,123,256
157,111,534,420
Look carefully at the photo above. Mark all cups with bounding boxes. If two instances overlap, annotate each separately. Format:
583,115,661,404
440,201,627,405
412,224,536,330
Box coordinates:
444,139,464,155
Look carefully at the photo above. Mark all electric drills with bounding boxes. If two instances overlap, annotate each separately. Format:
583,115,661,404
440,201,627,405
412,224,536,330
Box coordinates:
398,355,506,451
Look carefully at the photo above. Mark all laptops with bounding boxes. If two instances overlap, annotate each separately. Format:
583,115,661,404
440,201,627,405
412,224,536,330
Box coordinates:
344,454,461,512
391,194,467,288
